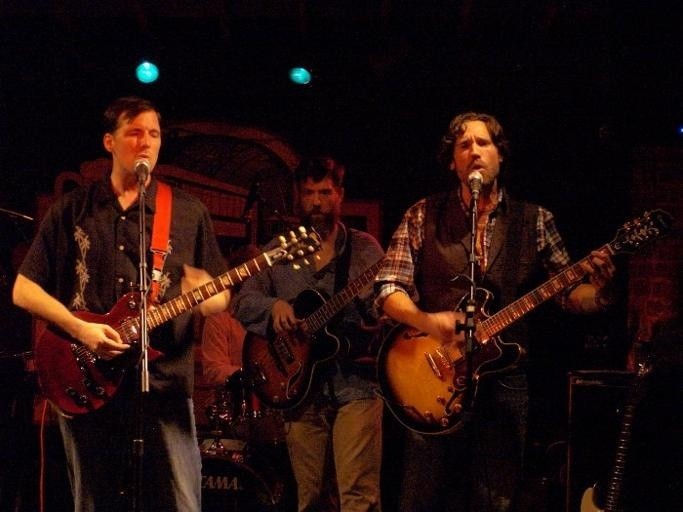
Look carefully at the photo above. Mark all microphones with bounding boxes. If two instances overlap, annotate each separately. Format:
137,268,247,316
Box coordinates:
134,158,150,185
242,179,261,224
467,170,483,201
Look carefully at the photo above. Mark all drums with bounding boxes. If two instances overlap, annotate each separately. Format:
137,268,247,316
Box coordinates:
170,440,284,512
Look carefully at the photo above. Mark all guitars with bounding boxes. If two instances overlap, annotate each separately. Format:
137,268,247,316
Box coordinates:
35,227,323,417
581,339,655,512
378,210,670,435
242,256,386,410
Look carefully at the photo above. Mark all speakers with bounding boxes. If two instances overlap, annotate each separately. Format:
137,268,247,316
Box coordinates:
200,438,248,512
566,369,638,512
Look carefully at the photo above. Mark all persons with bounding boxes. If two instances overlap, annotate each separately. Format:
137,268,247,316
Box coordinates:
201,244,262,386
12,98,232,512
373,112,618,512
231,160,385,512
